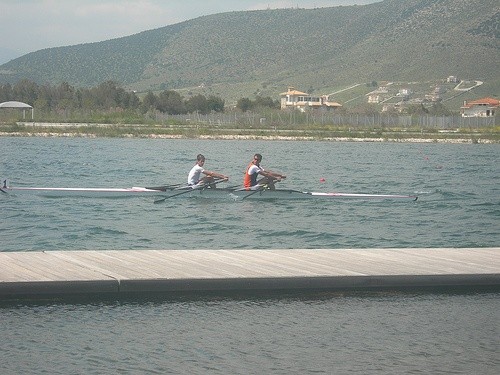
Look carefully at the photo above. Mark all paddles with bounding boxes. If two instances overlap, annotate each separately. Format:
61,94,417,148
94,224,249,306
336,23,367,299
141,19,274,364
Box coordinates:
241,181,280,199
153,178,228,203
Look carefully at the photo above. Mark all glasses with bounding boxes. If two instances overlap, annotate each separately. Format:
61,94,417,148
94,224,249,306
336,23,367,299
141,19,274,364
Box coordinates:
254,158,261,161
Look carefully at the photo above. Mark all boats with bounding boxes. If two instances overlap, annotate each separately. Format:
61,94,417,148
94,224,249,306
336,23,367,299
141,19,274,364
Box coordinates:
0,179,416,201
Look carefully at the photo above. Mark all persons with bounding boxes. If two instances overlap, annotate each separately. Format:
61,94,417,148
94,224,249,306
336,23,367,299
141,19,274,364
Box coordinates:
188,154,229,189
244,154,286,190
